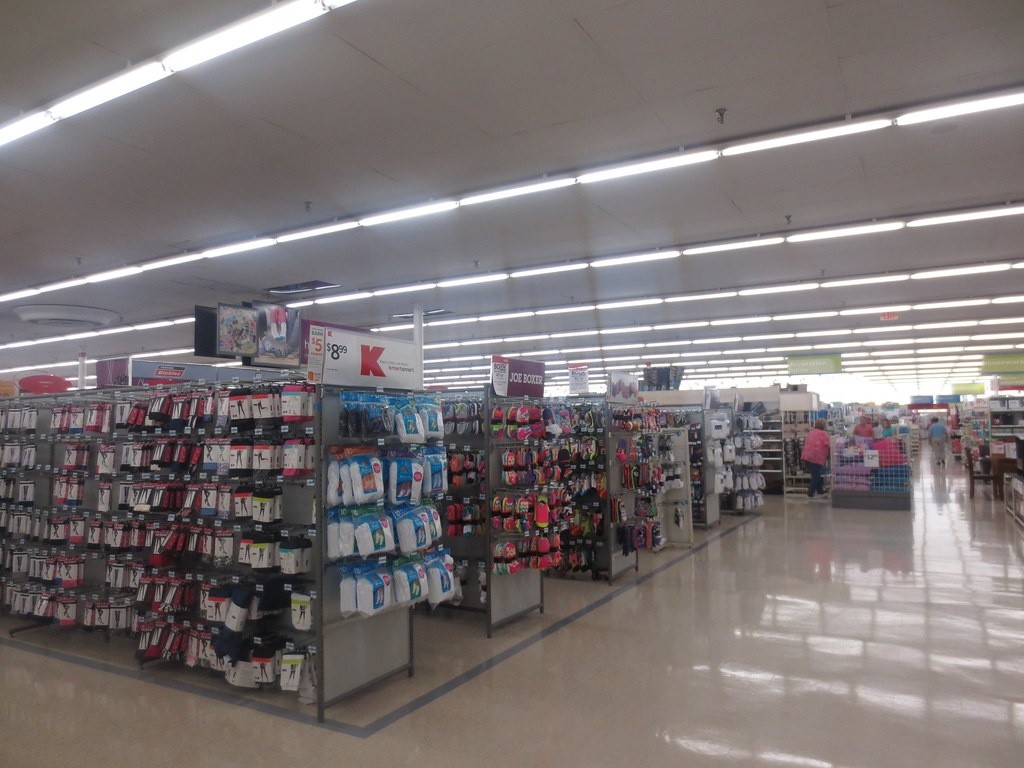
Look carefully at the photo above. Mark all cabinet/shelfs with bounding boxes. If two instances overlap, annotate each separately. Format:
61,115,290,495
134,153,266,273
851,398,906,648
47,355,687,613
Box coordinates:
416,386,825,639
947,388,1024,525
0,383,417,724
830,424,921,508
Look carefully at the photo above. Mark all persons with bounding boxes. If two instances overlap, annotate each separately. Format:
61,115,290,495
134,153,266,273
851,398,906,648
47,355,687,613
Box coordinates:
801,419,832,499
928,416,950,466
864,414,900,427
927,416,934,430
854,416,875,438
253,303,303,359
0,391,308,686
881,419,895,437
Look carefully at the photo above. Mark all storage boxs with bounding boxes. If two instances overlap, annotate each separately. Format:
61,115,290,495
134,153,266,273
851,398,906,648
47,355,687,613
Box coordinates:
0,397,141,633
